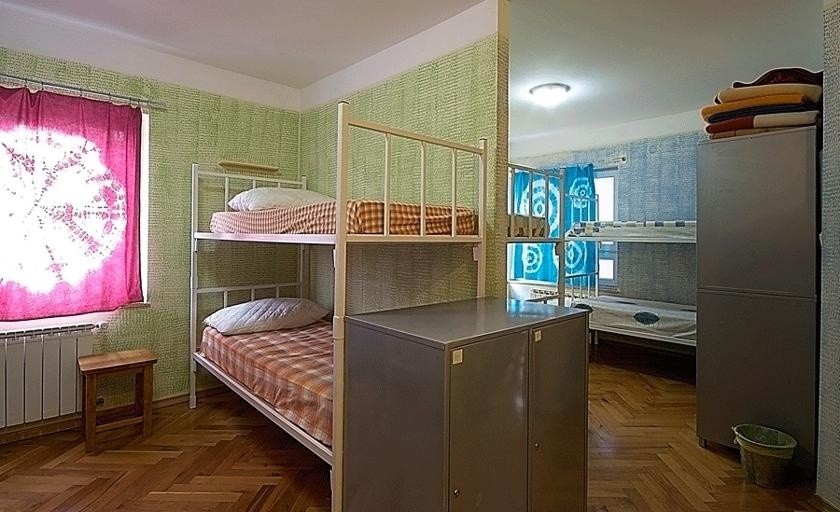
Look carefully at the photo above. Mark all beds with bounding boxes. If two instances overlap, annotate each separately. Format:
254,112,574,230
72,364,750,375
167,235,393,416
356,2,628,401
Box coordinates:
190,101,485,512
506,163,565,309
565,193,696,345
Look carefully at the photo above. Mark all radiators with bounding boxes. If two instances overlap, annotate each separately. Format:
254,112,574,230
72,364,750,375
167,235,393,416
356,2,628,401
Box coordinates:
0,321,108,430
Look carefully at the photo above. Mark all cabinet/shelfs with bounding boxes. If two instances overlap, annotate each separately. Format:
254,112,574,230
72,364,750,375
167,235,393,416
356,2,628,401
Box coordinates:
342,295,587,512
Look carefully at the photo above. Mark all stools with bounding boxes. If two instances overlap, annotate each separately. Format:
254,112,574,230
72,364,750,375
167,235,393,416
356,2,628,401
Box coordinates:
77,349,158,441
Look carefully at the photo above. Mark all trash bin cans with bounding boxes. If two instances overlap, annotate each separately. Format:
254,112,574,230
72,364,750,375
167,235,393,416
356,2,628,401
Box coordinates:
731,424,797,489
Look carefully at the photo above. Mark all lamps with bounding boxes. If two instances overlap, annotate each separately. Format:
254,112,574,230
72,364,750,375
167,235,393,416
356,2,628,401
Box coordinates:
530,83,571,108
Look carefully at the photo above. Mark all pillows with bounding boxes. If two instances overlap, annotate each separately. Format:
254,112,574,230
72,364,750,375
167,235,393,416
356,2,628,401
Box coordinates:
202,297,330,335
229,187,336,210
